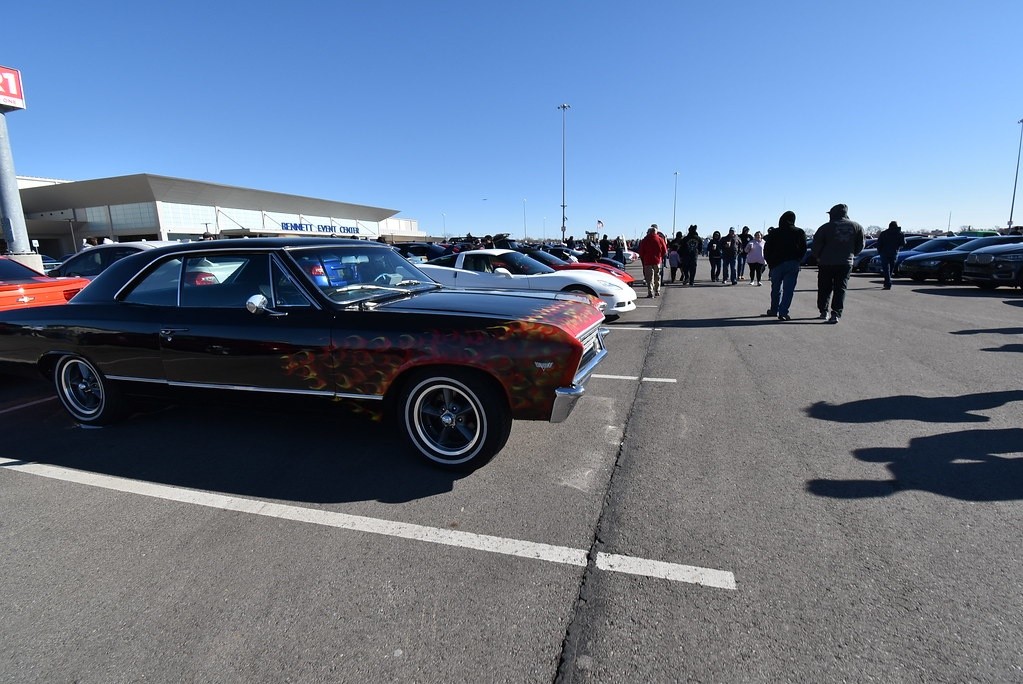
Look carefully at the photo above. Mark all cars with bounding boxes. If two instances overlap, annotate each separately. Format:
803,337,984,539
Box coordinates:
801,231,1023,289
0,236,609,470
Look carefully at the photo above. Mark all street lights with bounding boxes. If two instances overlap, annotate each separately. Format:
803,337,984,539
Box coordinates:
672,170,680,240
1009,118,1023,234
442,213,446,236
522,198,527,238
557,104,572,241
543,217,546,241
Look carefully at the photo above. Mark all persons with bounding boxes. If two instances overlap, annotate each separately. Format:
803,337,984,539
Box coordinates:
764,210,808,320
876,220,905,289
81,217,776,298
811,203,865,325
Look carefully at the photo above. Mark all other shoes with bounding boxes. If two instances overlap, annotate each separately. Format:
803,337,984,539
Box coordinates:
682,277,694,286
819,310,828,320
748,280,754,285
767,309,776,316
829,315,840,323
648,290,660,299
779,314,791,320
757,281,762,286
722,280,726,284
712,277,719,282
733,279,737,285
737,275,744,280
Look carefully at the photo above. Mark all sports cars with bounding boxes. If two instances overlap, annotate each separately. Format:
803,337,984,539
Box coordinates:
0,236,642,320
